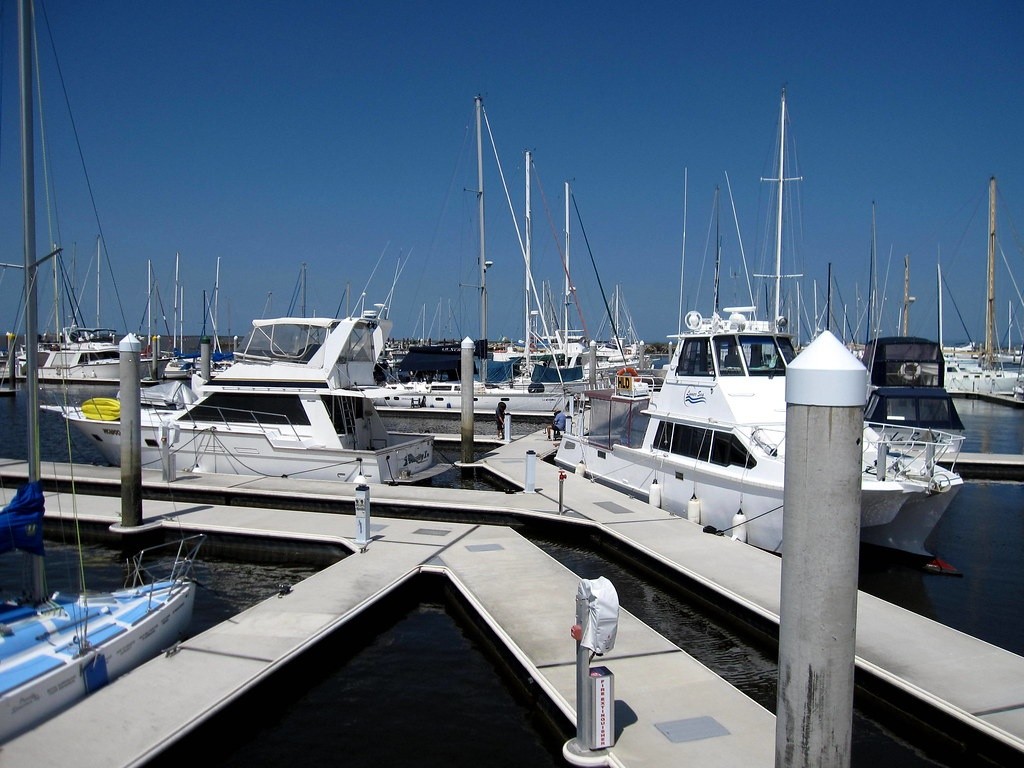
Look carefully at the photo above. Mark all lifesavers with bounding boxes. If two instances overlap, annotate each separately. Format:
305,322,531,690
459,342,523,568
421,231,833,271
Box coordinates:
615,369,642,382
900,362,921,381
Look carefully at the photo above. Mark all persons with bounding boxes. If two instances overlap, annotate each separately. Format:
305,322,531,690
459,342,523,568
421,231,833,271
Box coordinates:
545,408,566,441
495,402,506,440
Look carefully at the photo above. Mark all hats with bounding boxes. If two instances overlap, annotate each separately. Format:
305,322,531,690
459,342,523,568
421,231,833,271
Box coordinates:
498,401,506,410
554,407,561,412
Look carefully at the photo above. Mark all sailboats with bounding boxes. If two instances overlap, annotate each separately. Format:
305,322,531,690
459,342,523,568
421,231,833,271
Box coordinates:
0,0,211,744
1,85,1024,577
